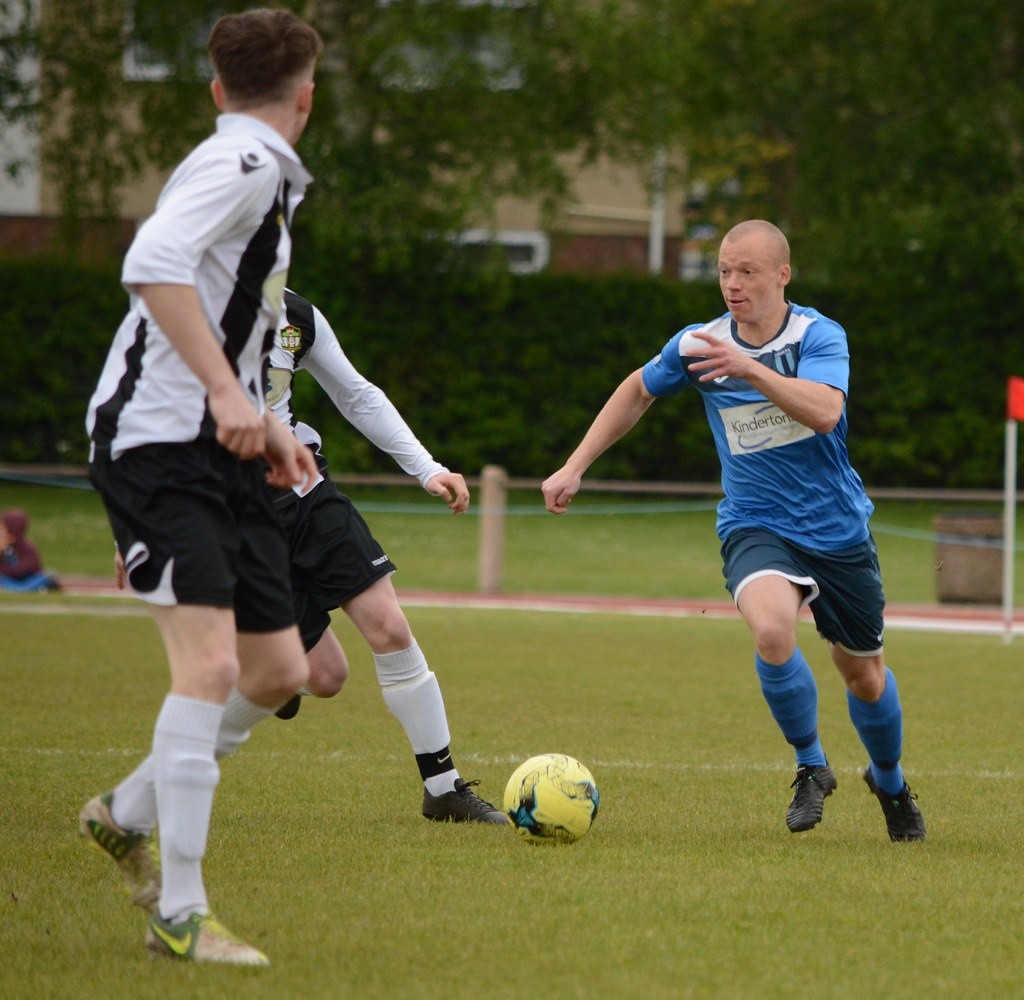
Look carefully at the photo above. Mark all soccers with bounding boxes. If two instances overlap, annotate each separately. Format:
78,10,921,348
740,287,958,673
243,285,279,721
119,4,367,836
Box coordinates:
503,754,601,849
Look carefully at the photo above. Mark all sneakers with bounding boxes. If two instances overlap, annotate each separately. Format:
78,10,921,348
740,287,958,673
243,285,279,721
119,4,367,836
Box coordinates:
422,777,507,825
786,753,837,832
148,910,271,970
80,792,162,912
276,694,301,720
862,766,926,841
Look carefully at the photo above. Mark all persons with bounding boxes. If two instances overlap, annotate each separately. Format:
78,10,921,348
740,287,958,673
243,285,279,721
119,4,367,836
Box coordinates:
77,7,512,967
540,219,926,842
0,509,66,593
